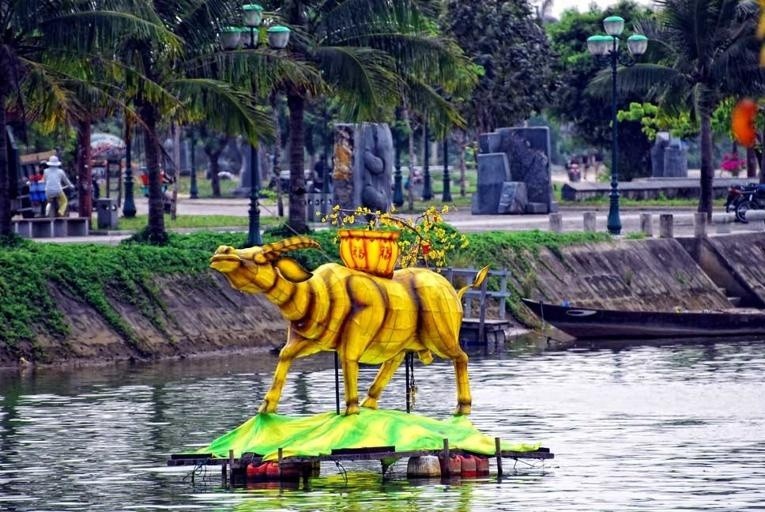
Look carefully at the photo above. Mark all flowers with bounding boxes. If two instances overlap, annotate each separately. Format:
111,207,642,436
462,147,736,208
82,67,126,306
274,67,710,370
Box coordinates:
313,197,469,275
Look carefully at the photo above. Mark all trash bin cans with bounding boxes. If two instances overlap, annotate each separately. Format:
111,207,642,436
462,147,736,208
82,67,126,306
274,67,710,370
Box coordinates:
97,198,116,228
308,192,332,222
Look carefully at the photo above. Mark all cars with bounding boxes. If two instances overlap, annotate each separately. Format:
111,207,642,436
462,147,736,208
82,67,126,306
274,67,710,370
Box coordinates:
725,182,765,225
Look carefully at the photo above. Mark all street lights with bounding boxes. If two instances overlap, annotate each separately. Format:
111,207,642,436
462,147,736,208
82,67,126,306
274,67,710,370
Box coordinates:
219,4,290,248
586,16,650,235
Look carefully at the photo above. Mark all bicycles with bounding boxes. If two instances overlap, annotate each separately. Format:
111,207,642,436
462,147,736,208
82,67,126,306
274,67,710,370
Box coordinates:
44,185,70,217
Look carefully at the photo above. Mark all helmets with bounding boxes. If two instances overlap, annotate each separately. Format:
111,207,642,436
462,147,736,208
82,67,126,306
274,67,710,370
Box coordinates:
39,160,49,168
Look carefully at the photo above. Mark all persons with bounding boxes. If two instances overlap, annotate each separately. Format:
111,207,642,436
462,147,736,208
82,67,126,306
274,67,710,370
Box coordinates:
39,159,59,217
567,159,581,182
40,155,75,216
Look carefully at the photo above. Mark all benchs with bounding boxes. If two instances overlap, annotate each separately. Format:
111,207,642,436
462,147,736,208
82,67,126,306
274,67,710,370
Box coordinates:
11,216,91,238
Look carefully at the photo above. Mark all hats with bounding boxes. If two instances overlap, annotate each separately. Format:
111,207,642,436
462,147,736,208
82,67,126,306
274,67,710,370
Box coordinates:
46,155,61,166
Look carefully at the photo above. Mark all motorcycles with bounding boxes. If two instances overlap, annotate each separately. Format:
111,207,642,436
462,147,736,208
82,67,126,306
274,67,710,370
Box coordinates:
567,163,580,181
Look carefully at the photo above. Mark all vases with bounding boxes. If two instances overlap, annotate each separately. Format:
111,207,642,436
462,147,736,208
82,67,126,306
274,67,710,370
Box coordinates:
335,225,401,278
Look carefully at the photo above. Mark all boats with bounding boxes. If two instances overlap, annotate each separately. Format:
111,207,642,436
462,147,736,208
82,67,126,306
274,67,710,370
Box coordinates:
521,298,765,340
